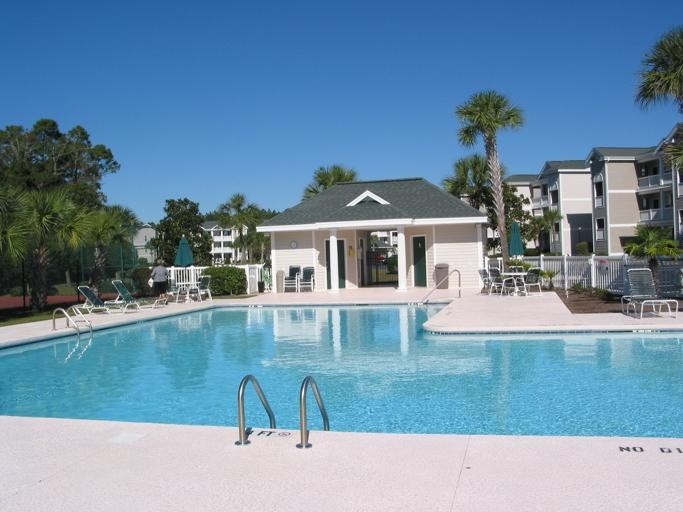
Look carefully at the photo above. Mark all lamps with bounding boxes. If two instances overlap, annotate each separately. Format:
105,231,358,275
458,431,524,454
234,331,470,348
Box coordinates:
620,267,679,319
103,280,142,316
194,276,212,302
298,266,314,292
283,265,301,292
478,266,543,297
74,285,112,316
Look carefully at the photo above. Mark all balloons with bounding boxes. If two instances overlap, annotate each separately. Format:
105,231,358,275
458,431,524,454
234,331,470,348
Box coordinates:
290,240,297,249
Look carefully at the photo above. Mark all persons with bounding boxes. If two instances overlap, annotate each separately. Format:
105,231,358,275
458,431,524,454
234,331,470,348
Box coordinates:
151,258,170,304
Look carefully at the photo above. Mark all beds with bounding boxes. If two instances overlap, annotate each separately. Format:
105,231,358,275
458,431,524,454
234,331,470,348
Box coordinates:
174,233,195,282
508,219,524,265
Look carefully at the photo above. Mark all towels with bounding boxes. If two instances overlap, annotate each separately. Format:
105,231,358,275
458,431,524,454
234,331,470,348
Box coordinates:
258,282,264,292
435,264,449,289
